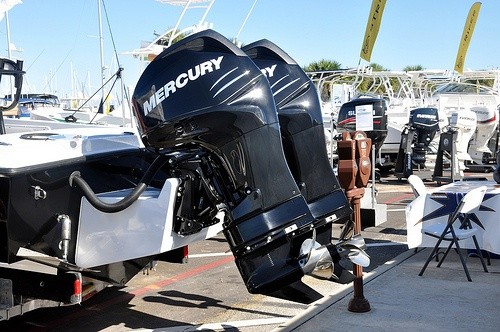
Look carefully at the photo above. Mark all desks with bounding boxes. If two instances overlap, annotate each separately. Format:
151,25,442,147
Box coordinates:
422,181,500,266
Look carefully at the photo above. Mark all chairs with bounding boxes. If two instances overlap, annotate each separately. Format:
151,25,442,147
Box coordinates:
418,186,488,283
407,175,426,198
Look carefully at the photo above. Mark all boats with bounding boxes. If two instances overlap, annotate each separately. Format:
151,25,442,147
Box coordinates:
0,0,499,308
